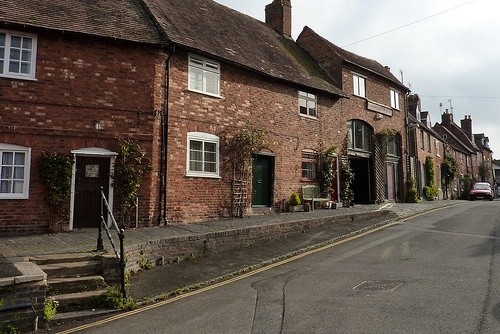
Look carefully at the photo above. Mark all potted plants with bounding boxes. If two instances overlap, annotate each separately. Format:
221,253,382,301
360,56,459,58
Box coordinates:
289,192,301,213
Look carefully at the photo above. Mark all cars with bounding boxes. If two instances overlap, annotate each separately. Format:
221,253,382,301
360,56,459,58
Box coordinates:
468,182,494,202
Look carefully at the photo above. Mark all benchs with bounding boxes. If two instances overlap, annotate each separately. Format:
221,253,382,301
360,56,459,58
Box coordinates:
302,184,332,212
